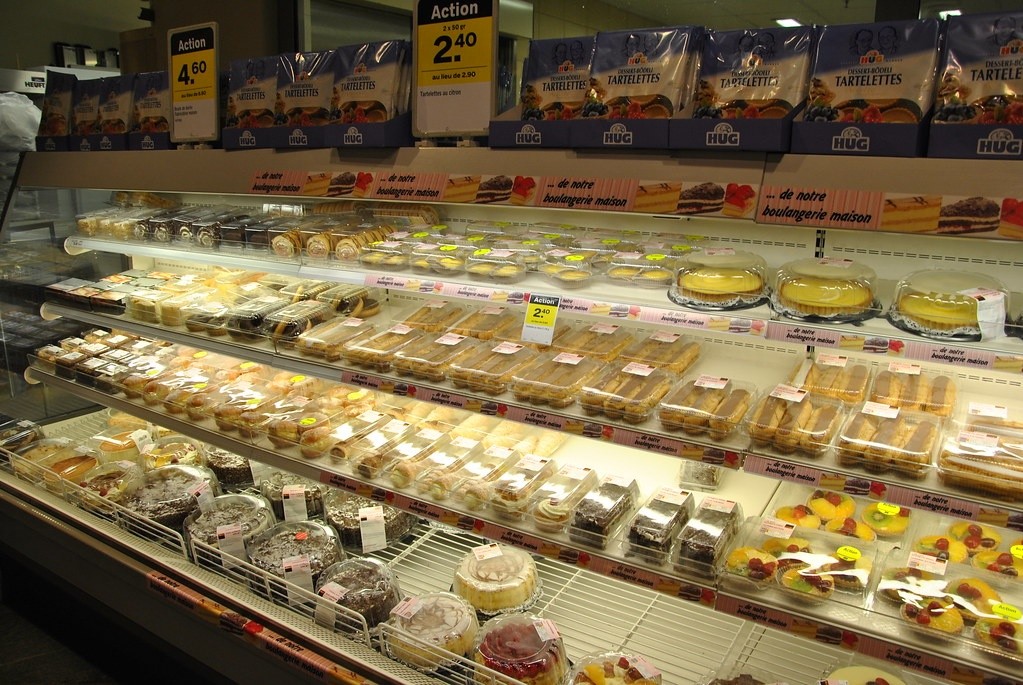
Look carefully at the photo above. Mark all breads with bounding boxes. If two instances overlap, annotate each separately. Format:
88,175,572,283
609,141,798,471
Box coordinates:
39,270,755,547
77,192,437,266
750,349,1023,505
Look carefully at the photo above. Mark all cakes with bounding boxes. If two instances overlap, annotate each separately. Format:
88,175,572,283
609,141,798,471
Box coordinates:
0,416,908,685
673,266,980,332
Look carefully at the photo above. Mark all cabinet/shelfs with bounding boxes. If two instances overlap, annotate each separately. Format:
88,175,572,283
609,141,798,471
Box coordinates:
0,185,1023,685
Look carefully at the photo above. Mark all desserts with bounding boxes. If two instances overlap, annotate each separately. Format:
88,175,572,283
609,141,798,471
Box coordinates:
878,192,1023,240
299,170,760,219
358,227,703,289
725,490,1023,663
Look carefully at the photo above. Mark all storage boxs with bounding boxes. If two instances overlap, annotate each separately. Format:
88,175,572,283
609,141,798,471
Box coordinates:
571,118,670,148
489,103,572,147
272,126,326,147
791,104,932,157
926,112,1023,159
670,96,809,152
222,127,272,148
129,132,171,150
68,135,96,150
326,109,413,147
95,133,129,150
35,136,68,151
